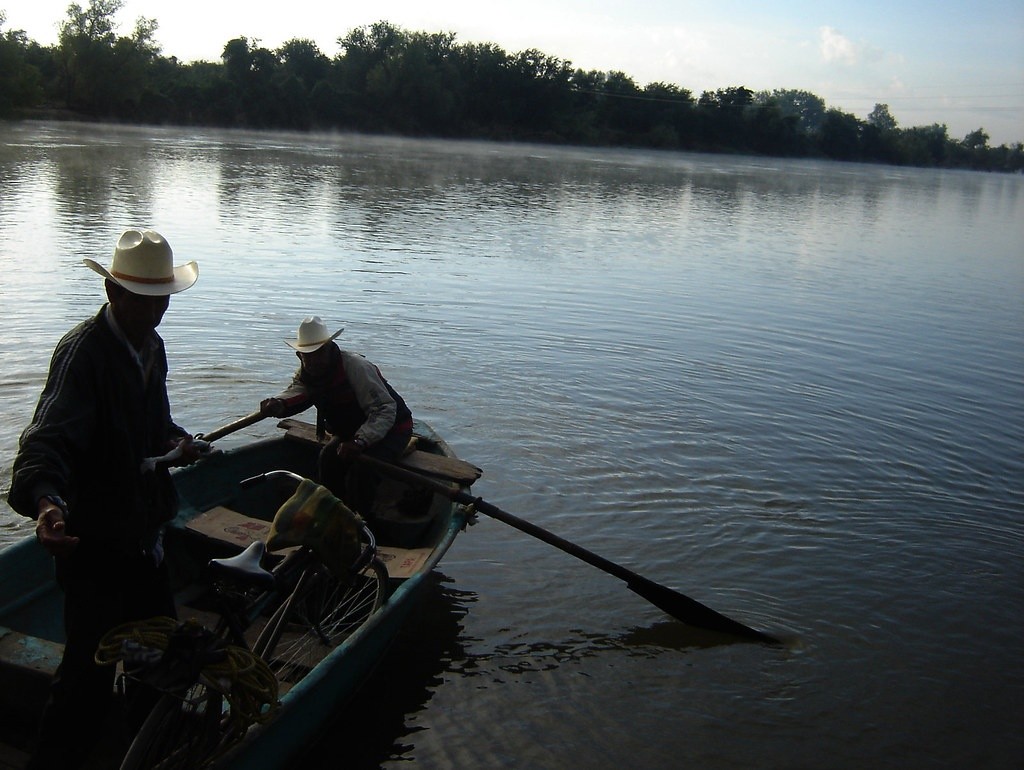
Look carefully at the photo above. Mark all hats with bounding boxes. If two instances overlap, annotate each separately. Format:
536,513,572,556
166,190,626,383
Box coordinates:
283,315,345,354
83,227,199,297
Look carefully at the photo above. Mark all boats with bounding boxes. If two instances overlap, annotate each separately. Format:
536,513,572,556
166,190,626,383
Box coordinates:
0,415,480,770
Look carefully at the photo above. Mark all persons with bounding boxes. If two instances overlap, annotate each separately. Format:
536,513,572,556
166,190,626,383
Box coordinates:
260,316,414,518
7,231,199,770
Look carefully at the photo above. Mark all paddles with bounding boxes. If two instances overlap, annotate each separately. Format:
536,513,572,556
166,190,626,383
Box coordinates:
197,409,268,443
318,436,761,641
